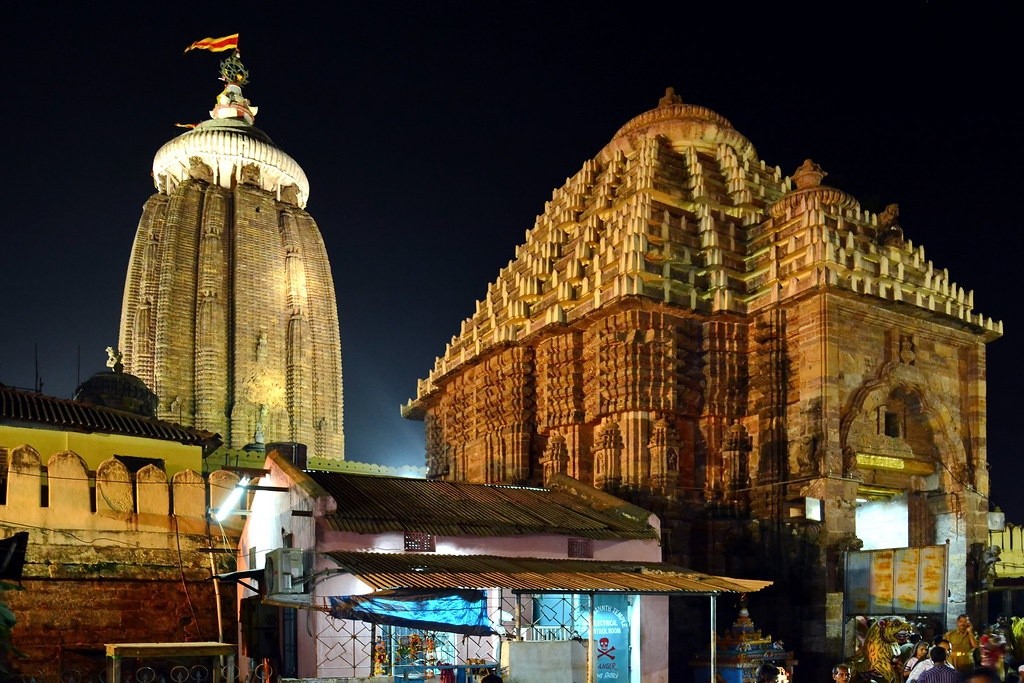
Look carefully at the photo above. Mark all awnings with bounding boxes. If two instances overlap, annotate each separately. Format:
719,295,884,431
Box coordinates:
322,551,774,593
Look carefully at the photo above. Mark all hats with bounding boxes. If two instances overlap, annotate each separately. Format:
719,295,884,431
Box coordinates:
755,663,780,677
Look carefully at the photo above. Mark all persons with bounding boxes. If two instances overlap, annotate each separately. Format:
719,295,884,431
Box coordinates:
832,664,851,683
845,608,1024,683
756,663,780,683
